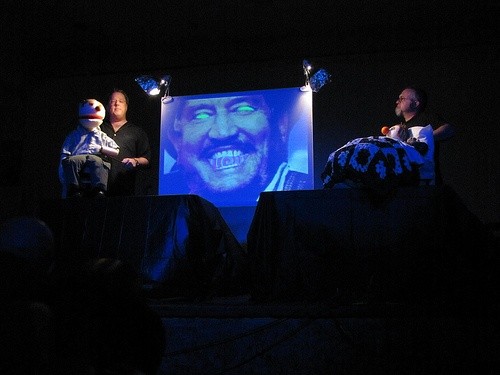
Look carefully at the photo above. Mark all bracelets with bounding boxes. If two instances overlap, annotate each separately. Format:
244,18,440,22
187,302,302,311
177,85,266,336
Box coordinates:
132,157,139,166
99,146,104,153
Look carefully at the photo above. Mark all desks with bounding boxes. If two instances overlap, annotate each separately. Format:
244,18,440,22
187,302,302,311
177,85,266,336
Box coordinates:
247,190,442,301
36,194,243,303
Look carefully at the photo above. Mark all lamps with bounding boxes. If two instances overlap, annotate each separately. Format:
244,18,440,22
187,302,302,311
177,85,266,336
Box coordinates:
301,58,312,91
159,75,172,104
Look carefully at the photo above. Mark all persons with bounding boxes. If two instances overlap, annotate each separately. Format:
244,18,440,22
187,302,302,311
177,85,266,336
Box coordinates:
162,95,309,207
99,89,152,196
0,215,168,375
391,87,457,140
59,99,120,198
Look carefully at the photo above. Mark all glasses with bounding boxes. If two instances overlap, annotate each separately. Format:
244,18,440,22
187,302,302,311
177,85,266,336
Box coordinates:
399,97,416,102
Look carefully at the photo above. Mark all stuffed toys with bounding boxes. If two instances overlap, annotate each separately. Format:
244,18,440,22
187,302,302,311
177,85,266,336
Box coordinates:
381,124,415,145
